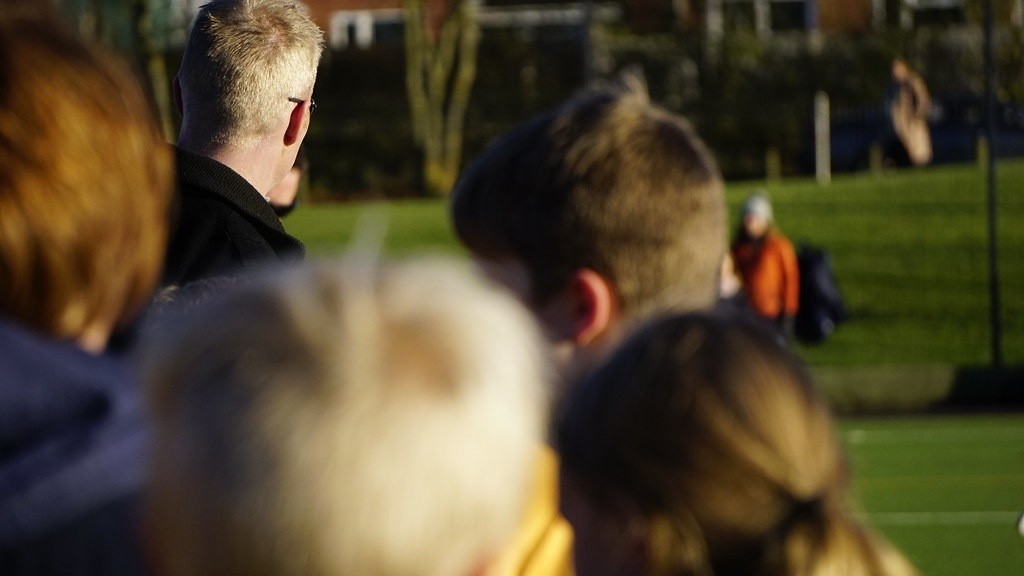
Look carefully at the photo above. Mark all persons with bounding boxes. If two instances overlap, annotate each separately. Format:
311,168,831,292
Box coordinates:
883,57,932,168
105,0,322,360
140,255,553,576
731,193,796,337
446,89,728,395
547,308,911,575
0,14,174,576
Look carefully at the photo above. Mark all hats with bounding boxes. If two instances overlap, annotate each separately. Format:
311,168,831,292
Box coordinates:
742,195,774,220
